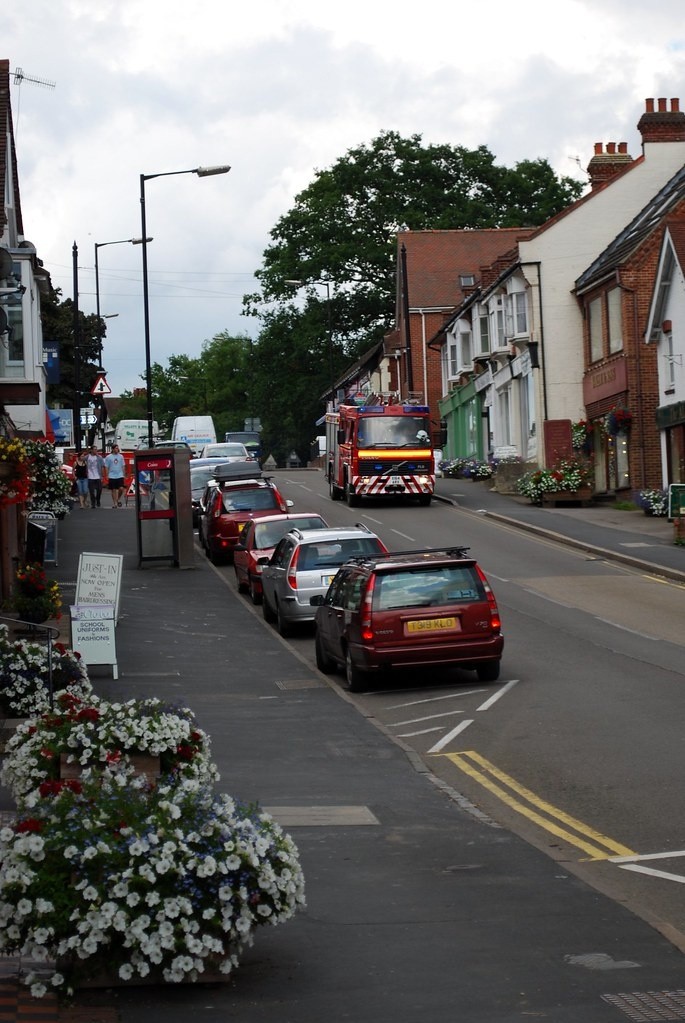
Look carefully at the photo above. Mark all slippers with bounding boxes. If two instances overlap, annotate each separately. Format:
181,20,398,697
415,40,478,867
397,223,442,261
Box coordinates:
112,505,117,508
117,501,122,507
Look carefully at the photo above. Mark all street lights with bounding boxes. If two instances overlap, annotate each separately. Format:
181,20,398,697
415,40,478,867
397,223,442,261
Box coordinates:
283,279,335,404
94,235,156,456
136,164,232,512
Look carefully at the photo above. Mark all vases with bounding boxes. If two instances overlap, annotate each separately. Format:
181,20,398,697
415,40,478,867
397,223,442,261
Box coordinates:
0,945,21,980
441,470,455,478
60,752,102,779
469,470,486,482
19,949,58,986
541,487,590,508
642,506,665,516
0,462,13,477
162,952,230,984
11,609,50,639
127,752,161,777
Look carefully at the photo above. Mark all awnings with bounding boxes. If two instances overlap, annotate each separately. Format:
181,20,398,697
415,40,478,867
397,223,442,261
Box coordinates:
31,409,64,444
316,412,338,426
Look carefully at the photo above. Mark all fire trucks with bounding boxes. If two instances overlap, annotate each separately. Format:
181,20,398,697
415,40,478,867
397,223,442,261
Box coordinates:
316,389,448,508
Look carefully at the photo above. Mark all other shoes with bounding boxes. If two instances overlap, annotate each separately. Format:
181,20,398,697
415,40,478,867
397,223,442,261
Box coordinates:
92,505,96,508
83,501,89,507
80,505,83,509
97,499,100,506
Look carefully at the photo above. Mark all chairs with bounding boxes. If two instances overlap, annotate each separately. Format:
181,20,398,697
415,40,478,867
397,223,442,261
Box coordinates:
335,542,363,557
255,493,270,507
306,547,319,561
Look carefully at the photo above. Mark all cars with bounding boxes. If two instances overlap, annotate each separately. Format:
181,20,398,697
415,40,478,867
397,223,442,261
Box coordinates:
232,512,341,605
148,438,254,530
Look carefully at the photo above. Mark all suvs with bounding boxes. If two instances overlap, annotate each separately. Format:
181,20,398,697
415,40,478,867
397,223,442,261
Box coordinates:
310,546,502,694
190,460,293,567
257,522,393,639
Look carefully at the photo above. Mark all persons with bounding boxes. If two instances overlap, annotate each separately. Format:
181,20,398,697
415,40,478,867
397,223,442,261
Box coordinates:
72,446,105,509
105,445,125,508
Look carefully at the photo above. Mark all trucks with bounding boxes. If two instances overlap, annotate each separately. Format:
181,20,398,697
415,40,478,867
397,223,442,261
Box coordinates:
223,430,266,470
169,414,217,458
113,420,160,451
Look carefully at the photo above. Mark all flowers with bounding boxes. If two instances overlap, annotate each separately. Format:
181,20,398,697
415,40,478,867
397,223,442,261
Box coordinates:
0,437,309,996
437,403,671,517
